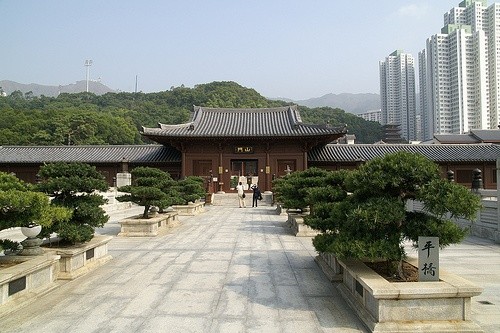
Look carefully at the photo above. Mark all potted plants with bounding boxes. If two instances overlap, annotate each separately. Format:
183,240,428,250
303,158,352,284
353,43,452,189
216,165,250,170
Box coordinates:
272,178,302,215
115,167,184,237
30,160,114,278
303,169,359,283
0,171,61,319
170,175,206,216
278,167,333,236
334,150,483,331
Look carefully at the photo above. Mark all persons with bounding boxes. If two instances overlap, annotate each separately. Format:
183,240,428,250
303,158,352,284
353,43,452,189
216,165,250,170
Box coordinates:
251,186,259,207
236,182,246,208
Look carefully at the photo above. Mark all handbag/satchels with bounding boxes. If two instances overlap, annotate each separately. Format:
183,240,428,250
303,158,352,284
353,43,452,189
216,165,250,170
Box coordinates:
258,195,263,201
242,193,245,198
